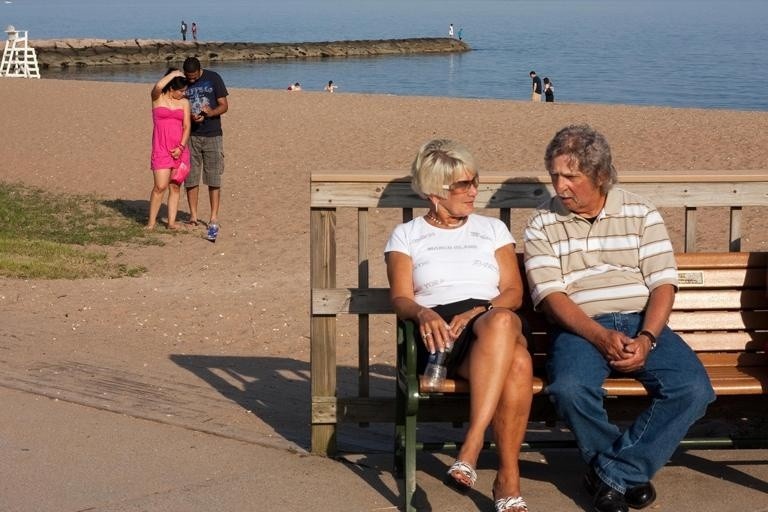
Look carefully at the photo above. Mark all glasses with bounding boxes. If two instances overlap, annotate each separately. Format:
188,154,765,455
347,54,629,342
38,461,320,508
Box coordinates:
442,171,480,195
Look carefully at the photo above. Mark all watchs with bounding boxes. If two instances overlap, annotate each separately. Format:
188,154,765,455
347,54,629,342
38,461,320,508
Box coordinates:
635,328,658,354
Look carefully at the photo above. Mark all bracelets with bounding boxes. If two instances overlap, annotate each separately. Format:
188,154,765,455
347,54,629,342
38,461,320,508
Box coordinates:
484,301,494,310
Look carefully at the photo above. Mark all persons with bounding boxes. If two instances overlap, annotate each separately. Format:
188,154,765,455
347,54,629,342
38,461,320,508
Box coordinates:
524,126,715,512
180,20,187,41
142,66,192,233
288,82,301,92
457,27,464,41
180,56,229,228
380,138,533,512
529,70,543,102
449,24,454,38
542,77,555,102
324,81,337,93
191,23,198,40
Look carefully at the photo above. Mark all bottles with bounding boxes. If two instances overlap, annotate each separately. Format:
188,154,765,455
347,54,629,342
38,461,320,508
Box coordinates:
424,339,453,395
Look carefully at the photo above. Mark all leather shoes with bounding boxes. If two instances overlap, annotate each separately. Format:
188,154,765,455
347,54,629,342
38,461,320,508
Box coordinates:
578,458,657,512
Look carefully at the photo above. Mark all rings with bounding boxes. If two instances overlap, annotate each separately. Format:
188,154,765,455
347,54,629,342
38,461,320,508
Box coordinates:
422,332,434,339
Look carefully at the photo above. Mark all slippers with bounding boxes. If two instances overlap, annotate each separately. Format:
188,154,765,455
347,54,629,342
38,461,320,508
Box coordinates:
445,460,477,494
493,488,528,512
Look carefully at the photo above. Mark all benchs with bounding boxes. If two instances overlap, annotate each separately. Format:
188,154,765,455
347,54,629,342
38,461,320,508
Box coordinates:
392,250,767,512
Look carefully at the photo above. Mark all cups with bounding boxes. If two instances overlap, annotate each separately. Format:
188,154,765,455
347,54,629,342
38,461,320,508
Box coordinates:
207,220,220,241
